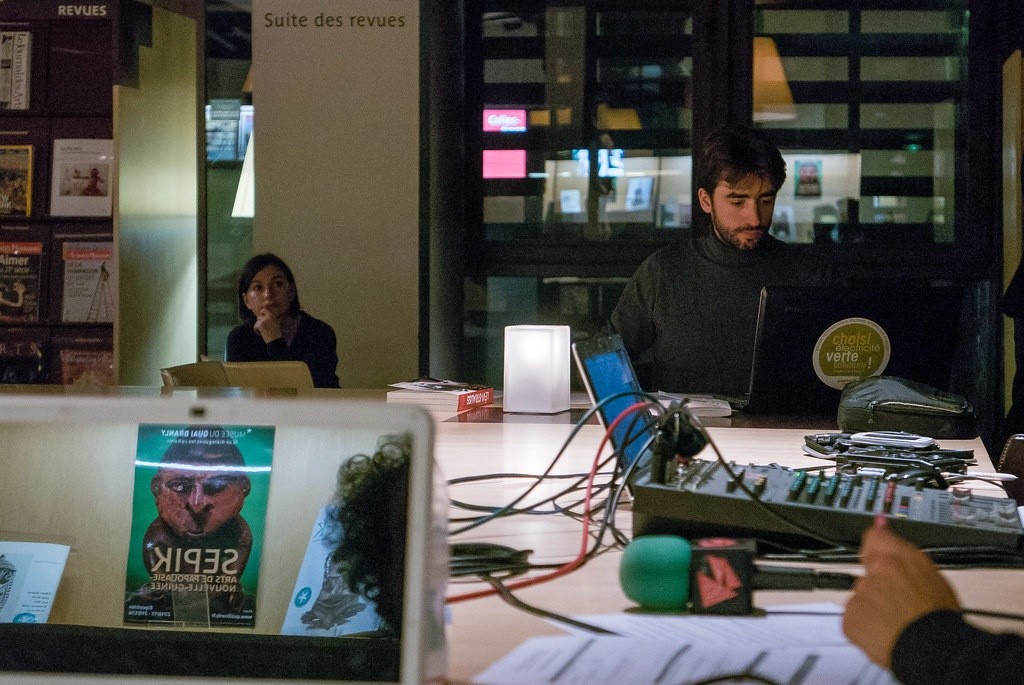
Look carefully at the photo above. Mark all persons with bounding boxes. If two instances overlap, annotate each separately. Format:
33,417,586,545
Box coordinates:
572,127,850,417
841,521,1023,685
223,251,342,388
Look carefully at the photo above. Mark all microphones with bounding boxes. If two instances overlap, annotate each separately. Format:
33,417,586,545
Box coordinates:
617,528,865,616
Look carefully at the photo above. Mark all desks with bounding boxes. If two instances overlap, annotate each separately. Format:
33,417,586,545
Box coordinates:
439,413,1024,685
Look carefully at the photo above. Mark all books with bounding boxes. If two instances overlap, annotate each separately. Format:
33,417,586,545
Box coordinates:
0,26,119,387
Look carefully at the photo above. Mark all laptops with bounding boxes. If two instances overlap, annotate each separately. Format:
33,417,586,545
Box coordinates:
712,282,965,432
2,395,437,685
571,331,663,505
